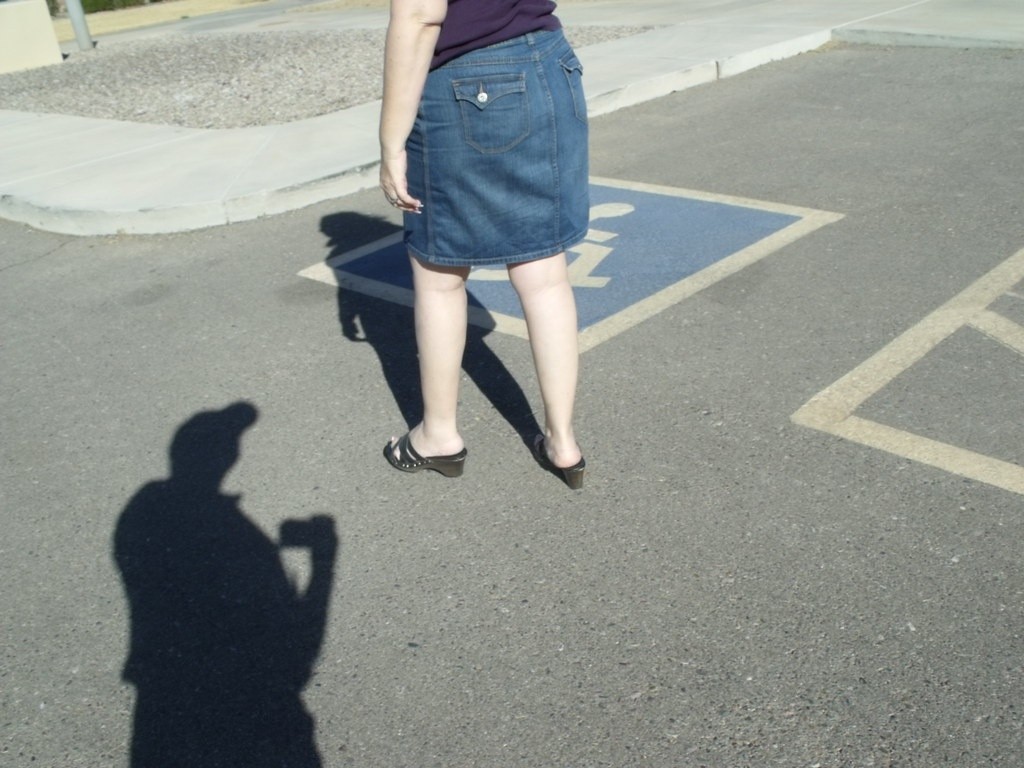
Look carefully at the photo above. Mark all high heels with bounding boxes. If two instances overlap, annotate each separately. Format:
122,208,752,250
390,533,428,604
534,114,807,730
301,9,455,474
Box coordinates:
384,431,467,477
534,438,586,489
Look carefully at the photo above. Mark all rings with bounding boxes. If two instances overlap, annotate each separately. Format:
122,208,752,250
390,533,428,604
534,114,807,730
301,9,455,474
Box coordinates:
389,199,398,203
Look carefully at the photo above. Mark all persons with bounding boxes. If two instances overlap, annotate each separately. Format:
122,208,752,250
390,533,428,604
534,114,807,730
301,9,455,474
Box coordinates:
376,0,590,490
